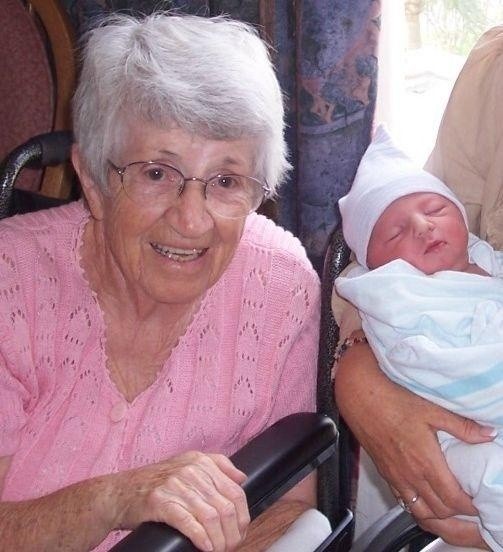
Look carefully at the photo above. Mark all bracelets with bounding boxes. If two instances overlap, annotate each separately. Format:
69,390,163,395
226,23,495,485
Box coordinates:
329,329,367,365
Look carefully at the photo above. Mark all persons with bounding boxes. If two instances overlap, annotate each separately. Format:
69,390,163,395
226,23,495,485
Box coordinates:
333,122,503,284
1,12,319,552
327,20,501,552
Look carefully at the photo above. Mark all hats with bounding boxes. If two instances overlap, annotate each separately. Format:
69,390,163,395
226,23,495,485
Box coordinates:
339,121,470,270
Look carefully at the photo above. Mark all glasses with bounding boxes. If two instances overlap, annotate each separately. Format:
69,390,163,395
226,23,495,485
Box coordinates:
106,154,269,219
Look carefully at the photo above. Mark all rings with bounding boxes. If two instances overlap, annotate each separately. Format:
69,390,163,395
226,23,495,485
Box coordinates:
405,493,421,512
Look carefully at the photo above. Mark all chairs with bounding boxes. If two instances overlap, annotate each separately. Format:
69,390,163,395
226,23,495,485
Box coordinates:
110,220,439,552
1,1,82,217
